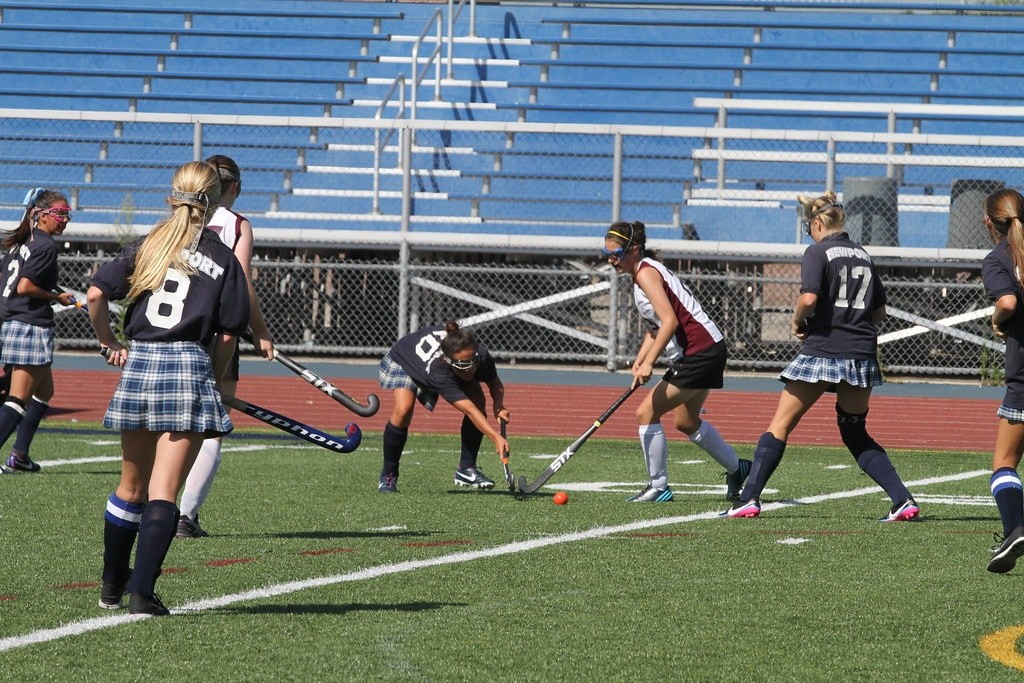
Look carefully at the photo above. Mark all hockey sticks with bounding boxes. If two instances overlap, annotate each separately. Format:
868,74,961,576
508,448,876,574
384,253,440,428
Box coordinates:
54,284,88,311
501,418,515,492
239,331,380,417
100,347,362,454
518,380,640,493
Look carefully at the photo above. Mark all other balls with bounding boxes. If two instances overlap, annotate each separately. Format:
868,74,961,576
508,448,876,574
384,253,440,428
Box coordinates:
554,492,568,505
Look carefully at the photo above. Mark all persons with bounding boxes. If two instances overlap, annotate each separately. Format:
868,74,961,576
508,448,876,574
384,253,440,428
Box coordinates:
718,192,919,521
378,321,510,494
0,187,74,474
604,221,753,502
982,188,1024,573
87,155,273,617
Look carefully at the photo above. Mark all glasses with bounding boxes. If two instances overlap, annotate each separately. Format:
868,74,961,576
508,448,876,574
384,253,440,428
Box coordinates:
601,245,631,259
801,219,813,236
238,179,241,197
451,352,482,369
49,206,74,222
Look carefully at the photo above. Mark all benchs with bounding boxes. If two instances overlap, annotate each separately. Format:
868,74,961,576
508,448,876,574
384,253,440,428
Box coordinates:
0,0,1023,274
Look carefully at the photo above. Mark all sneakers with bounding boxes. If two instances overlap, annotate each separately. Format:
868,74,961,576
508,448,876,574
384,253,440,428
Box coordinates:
878,497,919,522
719,496,761,518
1,447,41,475
624,480,673,503
175,513,209,538
455,466,496,491
719,457,753,501
98,567,133,609
987,526,1024,574
377,472,397,493
128,592,170,618
